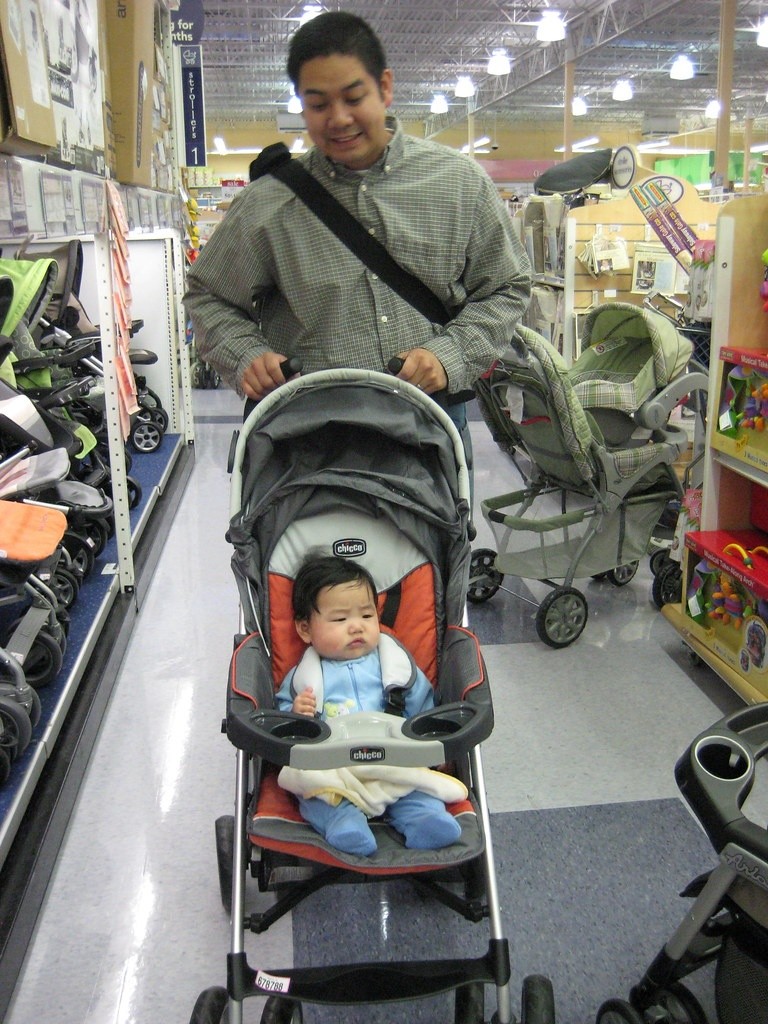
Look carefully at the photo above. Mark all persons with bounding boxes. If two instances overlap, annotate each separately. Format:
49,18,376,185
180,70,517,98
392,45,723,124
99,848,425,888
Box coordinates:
183,9,536,530
276,557,463,857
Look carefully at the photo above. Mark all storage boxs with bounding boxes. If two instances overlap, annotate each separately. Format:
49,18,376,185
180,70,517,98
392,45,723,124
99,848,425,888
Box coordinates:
105,1,179,194
1,0,116,180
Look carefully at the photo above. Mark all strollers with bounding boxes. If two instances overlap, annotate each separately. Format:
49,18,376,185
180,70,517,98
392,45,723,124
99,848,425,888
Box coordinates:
592,703,767,1024
461,292,698,648
2,241,168,801
191,354,559,1022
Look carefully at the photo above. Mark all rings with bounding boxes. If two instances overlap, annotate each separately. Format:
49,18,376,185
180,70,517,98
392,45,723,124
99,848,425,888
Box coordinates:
415,384,424,391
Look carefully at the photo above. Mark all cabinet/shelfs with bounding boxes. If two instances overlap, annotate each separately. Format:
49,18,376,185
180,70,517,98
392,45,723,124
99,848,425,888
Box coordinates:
658,190,768,707
